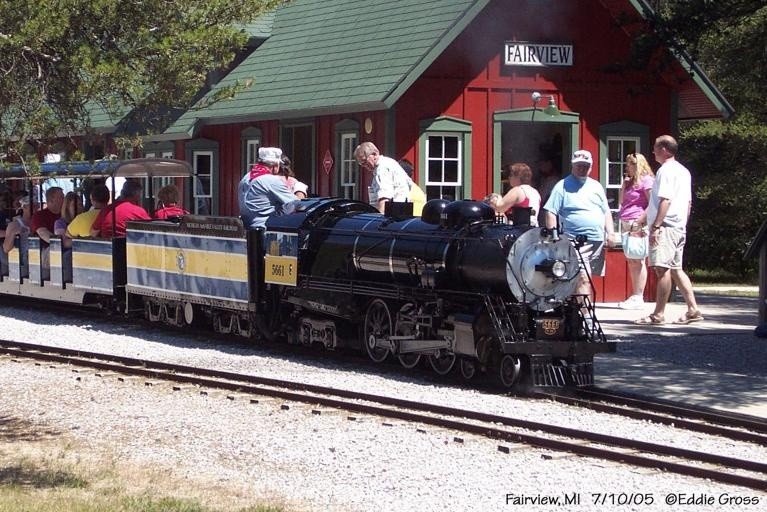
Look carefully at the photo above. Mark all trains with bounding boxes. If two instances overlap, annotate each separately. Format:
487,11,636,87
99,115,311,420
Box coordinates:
1,161,619,395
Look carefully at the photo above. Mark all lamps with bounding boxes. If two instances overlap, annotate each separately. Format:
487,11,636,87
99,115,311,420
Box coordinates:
531,91,561,115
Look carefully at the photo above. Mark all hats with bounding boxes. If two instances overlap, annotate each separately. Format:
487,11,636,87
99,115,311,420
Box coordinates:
258,147,285,165
570,149,592,165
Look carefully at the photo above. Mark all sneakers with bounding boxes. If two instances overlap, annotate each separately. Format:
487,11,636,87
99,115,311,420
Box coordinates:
618,295,644,310
586,316,598,337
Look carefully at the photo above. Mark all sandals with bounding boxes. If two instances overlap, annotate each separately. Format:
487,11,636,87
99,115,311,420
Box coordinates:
634,316,667,325
675,311,704,324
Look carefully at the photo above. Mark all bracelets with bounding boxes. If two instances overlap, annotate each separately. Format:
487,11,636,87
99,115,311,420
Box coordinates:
652,225,659,231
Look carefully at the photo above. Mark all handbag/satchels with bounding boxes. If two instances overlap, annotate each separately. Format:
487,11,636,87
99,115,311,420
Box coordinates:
621,222,649,260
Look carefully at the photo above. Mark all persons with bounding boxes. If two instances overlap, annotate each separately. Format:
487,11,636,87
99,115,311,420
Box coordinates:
542,150,615,333
353,142,411,214
618,153,655,310
276,156,308,199
537,158,561,227
237,147,301,230
1,179,191,281
399,159,427,217
489,163,542,228
634,135,703,325
